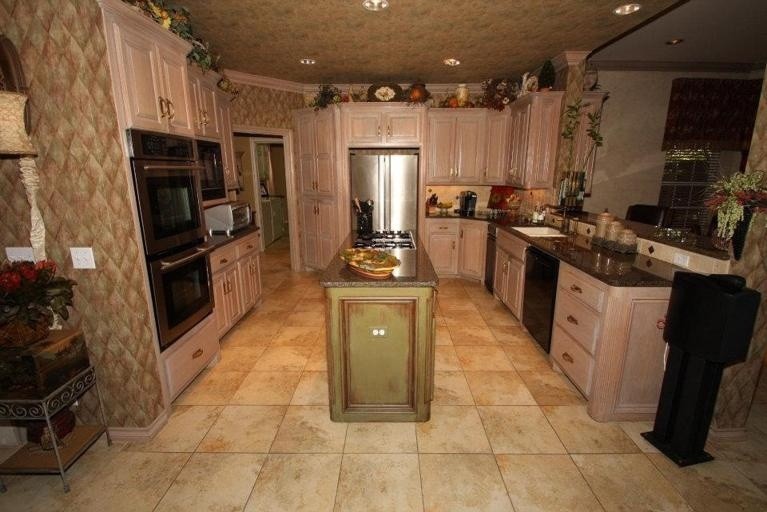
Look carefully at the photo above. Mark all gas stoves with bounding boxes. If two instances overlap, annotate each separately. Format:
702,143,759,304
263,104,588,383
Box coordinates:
351,230,415,249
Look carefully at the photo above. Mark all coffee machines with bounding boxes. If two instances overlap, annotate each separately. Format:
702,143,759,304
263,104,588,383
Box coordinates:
460,191,476,216
349,155,417,229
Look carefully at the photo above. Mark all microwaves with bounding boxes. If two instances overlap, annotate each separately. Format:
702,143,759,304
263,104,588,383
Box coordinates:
206,200,254,236
195,145,223,192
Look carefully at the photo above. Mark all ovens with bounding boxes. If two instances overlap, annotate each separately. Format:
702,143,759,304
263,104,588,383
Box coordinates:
131,154,215,349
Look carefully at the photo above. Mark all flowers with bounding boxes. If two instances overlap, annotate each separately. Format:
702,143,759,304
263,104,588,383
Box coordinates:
705,171,767,242
0,260,77,329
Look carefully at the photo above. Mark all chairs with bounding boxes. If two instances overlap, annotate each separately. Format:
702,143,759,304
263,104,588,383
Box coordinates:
624,204,667,226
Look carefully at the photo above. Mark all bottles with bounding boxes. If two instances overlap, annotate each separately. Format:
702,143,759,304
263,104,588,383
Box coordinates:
593,213,636,245
526,205,545,223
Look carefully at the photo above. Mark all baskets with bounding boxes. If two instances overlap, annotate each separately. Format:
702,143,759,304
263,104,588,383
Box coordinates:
339,245,401,280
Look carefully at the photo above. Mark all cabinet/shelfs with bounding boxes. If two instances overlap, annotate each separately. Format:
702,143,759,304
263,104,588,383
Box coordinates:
0,329,114,494
424,216,459,284
298,198,341,272
506,89,568,189
210,241,244,340
461,221,487,282
550,255,709,426
160,315,222,406
566,88,610,196
495,227,530,318
103,3,195,140
237,229,264,313
488,112,510,185
186,62,224,139
213,89,241,187
295,113,338,196
426,110,487,182
342,107,424,142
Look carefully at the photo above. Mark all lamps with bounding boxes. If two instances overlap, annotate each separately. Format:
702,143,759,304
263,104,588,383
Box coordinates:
0,87,37,159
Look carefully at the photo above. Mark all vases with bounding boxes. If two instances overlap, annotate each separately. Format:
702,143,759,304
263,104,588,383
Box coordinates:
0,308,54,346
456,83,470,108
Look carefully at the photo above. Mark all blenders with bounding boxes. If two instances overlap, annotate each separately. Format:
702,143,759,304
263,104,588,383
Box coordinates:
557,171,585,213
